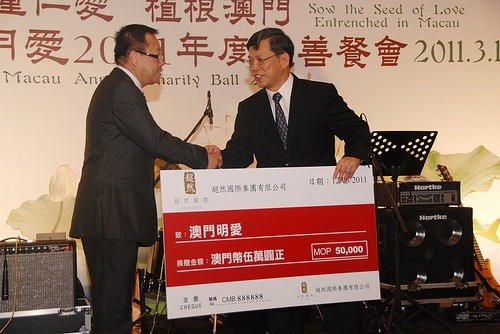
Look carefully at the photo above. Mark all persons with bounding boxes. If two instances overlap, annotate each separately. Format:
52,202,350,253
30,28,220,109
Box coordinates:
205,27,372,334
69,23,220,334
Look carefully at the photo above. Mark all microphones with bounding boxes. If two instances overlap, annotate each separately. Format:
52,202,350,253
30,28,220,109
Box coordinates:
1,260,9,300
207,91,213,124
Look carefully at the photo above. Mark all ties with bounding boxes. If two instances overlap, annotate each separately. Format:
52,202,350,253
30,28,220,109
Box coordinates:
272,91,287,151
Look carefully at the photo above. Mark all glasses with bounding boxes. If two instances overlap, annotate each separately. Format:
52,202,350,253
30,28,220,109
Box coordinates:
128,49,163,62
246,52,283,67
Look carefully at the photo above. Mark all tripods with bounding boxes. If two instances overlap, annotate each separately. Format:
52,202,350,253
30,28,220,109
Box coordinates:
365,131,452,334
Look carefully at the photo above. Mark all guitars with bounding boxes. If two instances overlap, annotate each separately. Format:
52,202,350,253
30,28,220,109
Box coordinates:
436,163,500,310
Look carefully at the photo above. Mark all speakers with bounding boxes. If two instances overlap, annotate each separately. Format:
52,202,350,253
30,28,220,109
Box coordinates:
377,206,477,298
0,239,76,314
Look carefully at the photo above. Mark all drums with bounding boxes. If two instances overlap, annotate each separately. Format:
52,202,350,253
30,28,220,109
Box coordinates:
144,228,166,302
